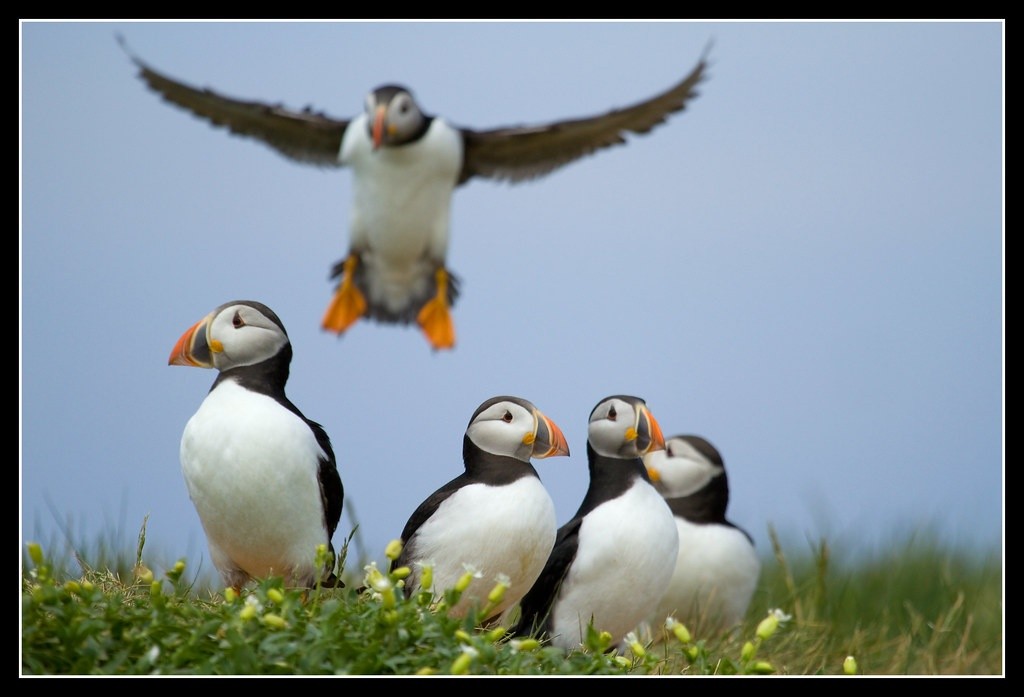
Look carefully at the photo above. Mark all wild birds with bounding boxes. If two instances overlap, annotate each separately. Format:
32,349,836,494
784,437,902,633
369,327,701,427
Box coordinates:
167,299,345,598
386,394,761,647
113,32,715,352
